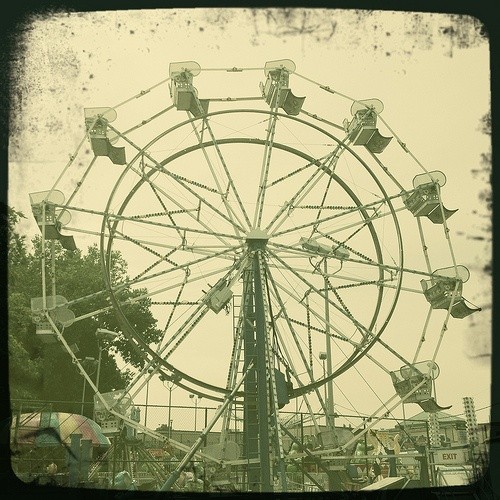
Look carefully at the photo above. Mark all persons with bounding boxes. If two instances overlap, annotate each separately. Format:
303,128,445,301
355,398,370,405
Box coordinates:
16,433,433,491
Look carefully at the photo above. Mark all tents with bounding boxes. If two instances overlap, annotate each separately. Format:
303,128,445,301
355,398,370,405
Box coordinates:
395,410,473,467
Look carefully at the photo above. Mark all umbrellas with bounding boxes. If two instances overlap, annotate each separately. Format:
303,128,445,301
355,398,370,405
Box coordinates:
10,410,114,450
